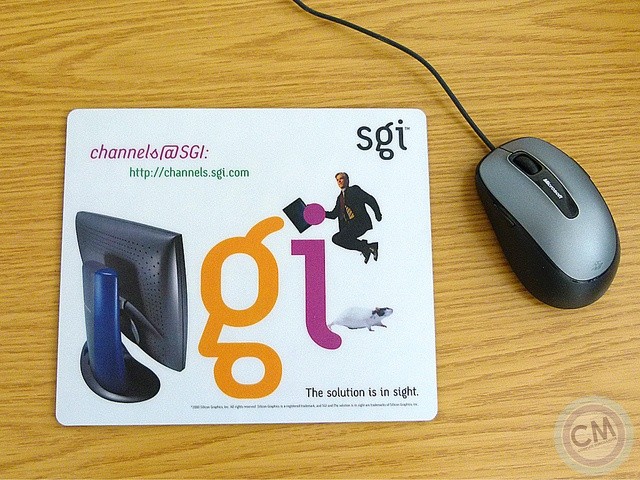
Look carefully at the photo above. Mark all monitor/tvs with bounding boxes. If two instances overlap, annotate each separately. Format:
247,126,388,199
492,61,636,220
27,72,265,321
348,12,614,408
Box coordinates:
74,211,188,404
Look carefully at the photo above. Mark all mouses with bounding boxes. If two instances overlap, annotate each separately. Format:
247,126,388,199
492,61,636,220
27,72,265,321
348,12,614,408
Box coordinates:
474,136,621,310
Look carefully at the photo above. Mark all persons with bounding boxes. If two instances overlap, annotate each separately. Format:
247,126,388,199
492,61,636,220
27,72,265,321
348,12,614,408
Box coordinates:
325,172,382,264
